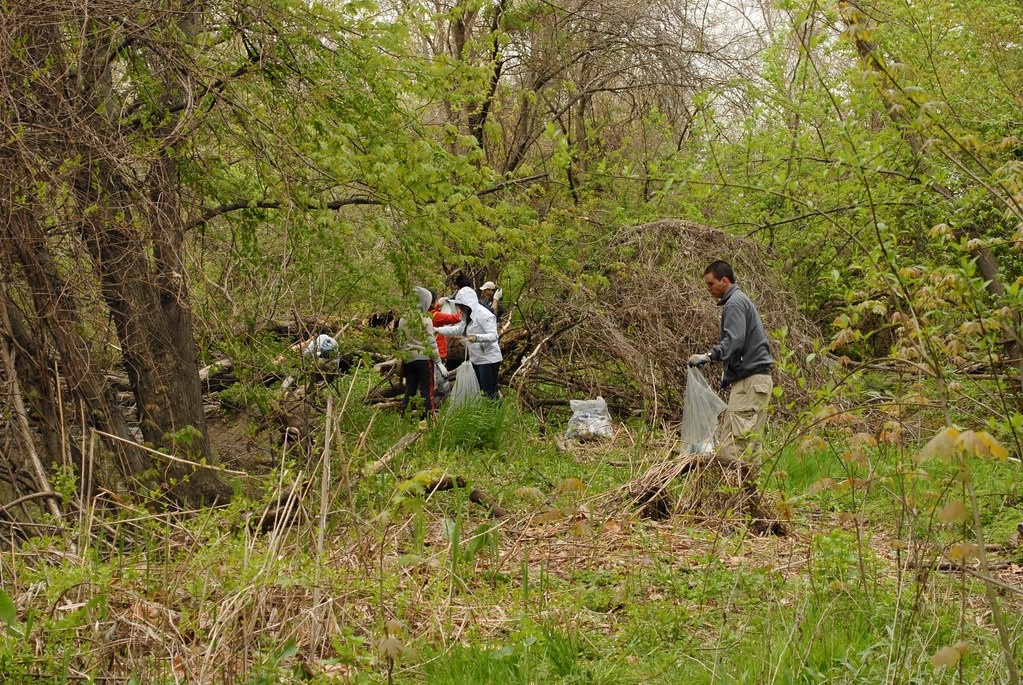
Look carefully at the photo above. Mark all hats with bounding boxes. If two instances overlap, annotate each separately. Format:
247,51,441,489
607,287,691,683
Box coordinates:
479,281,495,289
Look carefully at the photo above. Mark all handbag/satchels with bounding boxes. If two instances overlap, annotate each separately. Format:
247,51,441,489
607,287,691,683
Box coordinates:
450,339,482,405
396,357,405,377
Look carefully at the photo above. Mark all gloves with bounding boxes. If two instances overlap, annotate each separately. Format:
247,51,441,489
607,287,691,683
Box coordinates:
438,296,449,306
437,362,449,376
493,287,502,301
688,352,711,368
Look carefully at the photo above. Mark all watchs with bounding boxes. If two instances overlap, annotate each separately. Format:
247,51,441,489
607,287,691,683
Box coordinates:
492,297,499,302
708,352,713,365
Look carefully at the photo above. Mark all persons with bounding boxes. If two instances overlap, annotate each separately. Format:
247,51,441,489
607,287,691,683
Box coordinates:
450,274,503,317
479,281,504,323
688,260,774,490
397,286,449,421
298,334,342,406
427,289,464,371
433,285,503,413
